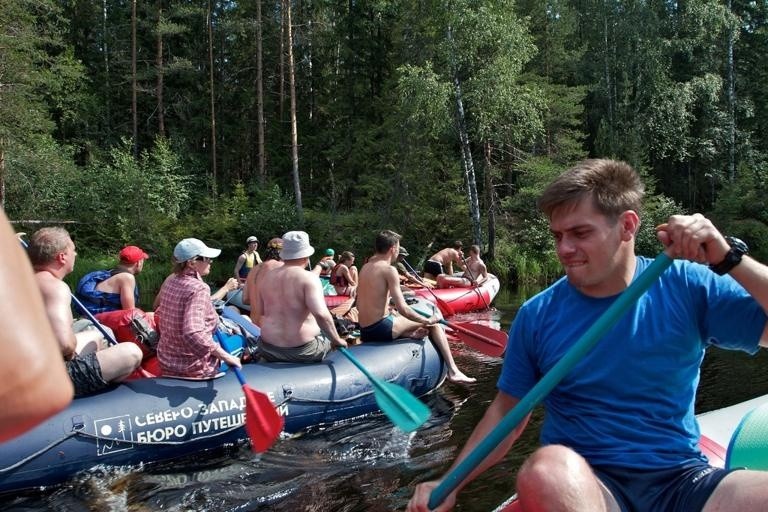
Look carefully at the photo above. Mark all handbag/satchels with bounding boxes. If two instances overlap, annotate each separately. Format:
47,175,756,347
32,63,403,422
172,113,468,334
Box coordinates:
74,269,115,313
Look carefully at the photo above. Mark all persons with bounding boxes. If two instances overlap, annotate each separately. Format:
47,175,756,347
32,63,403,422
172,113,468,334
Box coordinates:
26,227,145,401
75,246,150,320
155,237,242,377
232,231,491,386
399,157,768,511
0,208,79,452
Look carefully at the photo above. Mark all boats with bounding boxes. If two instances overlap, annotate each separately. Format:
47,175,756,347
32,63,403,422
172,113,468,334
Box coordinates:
226,277,340,314
0,296,450,489
492,392,767,511
323,270,501,324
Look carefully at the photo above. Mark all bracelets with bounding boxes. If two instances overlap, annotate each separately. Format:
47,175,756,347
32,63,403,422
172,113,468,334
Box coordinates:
703,233,748,276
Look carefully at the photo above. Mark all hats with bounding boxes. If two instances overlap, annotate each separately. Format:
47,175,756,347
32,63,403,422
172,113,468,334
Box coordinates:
279,231,315,260
246,235,260,243
326,249,334,255
174,238,222,263
120,247,149,265
265,238,282,254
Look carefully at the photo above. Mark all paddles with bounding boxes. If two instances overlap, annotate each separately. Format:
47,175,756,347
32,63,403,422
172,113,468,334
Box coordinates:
403,262,453,319
215,327,283,452
337,346,430,431
410,307,508,358
